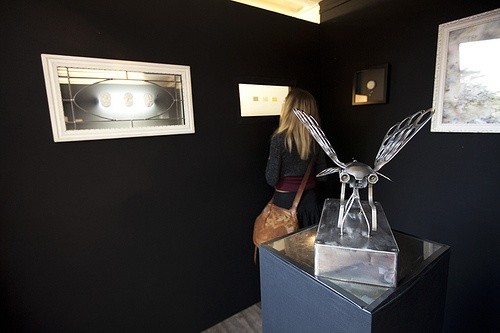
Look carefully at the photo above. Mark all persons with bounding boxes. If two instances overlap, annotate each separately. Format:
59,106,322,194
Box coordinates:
264,88,325,230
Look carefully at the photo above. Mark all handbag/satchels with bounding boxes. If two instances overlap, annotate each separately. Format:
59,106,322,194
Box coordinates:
253,200,299,249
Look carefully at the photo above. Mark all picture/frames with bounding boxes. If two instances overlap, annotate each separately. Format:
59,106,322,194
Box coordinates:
41,52,196,143
351,63,389,106
430,8,500,134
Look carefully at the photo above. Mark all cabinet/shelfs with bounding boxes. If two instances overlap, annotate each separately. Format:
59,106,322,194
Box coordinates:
259,219,451,333
